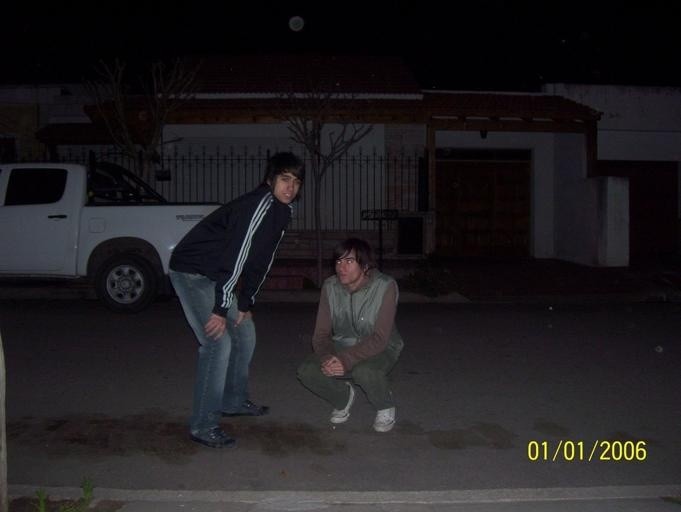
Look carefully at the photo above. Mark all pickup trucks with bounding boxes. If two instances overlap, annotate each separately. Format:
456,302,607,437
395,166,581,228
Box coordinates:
0,157,224,315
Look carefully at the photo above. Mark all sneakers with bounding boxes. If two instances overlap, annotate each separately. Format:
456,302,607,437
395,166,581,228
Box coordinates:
330,383,353,424
373,407,395,431
189,427,235,447
223,401,263,416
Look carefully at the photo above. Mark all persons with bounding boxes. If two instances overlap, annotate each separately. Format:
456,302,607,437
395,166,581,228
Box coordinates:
167,151,306,450
297,240,404,433
